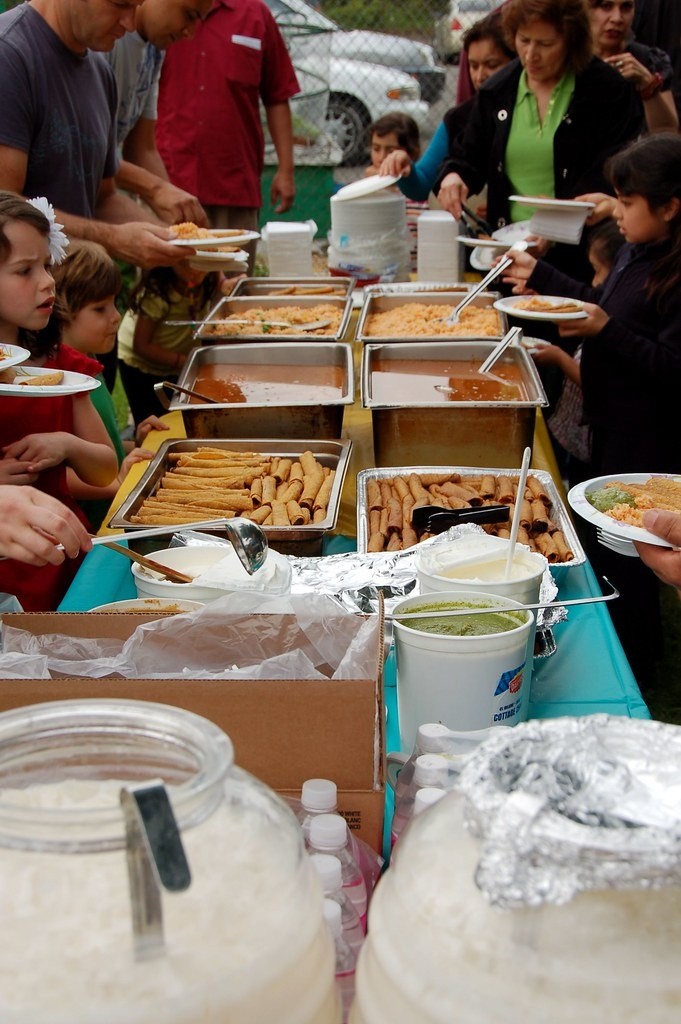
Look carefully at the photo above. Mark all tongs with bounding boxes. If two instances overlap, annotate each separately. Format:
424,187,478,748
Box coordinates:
434,184,494,239
413,505,510,534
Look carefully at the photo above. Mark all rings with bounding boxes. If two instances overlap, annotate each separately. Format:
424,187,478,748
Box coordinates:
615,60,624,67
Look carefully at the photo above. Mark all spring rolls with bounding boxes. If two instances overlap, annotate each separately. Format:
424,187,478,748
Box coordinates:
363,472,575,564
130,447,336,526
20,371,64,386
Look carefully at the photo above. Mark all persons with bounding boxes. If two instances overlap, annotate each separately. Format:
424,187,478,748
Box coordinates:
376,0,681,594
367,110,429,202
0,187,171,615
0,0,302,446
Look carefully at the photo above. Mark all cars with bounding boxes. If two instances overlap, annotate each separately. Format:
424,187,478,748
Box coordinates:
261,0,449,106
432,0,508,66
258,34,428,168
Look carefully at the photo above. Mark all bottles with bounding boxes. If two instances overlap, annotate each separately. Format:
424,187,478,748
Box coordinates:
390,724,459,868
310,854,365,1024
0,698,337,1024
297,779,366,938
346,718,681,1024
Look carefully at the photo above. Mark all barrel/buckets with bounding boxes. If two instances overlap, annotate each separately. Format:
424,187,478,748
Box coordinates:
417,538,546,723
86,599,206,612
131,547,237,605
392,591,534,756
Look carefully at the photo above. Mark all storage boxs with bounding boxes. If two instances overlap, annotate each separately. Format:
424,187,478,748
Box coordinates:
0,590,387,861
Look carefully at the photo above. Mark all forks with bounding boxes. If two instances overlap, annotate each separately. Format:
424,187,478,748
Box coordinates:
597,527,640,557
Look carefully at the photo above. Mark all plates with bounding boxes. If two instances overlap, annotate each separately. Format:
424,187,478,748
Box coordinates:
493,295,590,321
521,337,552,354
0,343,31,371
196,248,250,262
567,473,681,548
492,219,541,244
330,173,410,283
0,366,101,397
509,195,596,212
470,246,512,270
169,229,260,248
456,235,538,249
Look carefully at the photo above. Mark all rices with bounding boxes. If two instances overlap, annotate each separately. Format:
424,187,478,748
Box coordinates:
208,305,344,337
364,302,499,337
